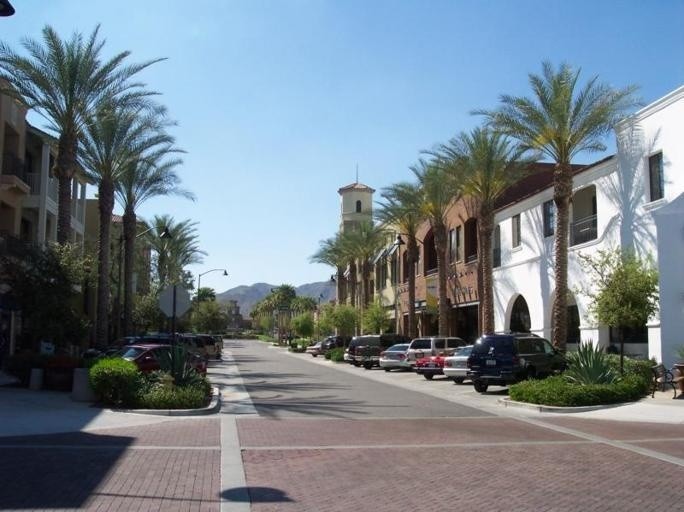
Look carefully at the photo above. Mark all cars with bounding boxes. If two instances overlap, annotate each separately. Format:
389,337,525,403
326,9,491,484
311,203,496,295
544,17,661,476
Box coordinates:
303,332,572,394
105,326,226,377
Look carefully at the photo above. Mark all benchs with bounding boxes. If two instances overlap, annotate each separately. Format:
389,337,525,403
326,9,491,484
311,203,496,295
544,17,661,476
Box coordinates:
648,362,683,400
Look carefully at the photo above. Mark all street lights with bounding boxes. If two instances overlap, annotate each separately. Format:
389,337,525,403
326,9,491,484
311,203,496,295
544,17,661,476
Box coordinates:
117,224,171,333
198,266,228,289
391,234,444,318
